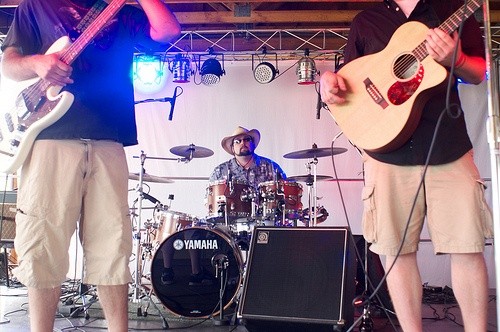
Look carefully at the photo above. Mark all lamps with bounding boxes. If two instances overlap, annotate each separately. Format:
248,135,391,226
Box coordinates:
136,53,162,87
251,47,279,84
168,54,193,84
198,51,225,87
296,55,318,85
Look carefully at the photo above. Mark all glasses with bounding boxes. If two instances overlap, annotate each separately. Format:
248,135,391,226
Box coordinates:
233,137,252,144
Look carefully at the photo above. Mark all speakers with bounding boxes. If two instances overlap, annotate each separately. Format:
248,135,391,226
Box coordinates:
0,191,18,242
236,226,358,331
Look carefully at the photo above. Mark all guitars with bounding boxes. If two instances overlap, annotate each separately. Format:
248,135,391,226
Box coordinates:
0,0,126,175
322,0,484,152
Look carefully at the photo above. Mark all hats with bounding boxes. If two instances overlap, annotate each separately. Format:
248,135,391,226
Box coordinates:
221,126,260,155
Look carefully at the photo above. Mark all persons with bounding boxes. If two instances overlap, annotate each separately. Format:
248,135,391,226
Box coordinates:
1,0,180,332
320,0,490,332
208,126,287,189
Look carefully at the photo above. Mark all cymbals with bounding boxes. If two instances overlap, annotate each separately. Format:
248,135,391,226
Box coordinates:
127,172,173,184
287,174,333,181
283,146,348,159
169,144,214,158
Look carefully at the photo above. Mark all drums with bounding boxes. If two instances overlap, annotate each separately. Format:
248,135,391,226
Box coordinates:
151,209,214,248
255,180,303,217
149,224,245,321
205,181,258,224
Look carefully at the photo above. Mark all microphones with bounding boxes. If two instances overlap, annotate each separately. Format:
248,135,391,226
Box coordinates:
169,87,177,120
316,84,320,119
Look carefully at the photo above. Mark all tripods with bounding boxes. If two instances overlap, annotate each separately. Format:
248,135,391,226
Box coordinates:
63,150,193,328
